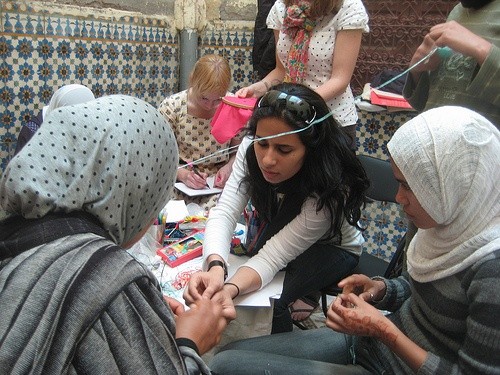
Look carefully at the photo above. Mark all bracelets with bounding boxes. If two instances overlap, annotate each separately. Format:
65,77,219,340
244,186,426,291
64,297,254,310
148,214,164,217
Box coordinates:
207,260,228,282
224,282,240,300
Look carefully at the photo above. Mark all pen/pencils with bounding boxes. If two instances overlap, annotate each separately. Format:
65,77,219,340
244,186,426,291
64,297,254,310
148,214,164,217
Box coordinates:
186,158,212,191
154,213,167,225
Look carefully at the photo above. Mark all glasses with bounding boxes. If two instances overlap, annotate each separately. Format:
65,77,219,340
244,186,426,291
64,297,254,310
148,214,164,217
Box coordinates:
258,89,317,124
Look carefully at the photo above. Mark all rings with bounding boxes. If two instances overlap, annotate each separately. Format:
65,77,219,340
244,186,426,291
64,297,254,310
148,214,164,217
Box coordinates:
366,290,373,299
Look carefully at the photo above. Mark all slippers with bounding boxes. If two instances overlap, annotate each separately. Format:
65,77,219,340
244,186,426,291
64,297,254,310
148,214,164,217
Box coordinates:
288,297,320,322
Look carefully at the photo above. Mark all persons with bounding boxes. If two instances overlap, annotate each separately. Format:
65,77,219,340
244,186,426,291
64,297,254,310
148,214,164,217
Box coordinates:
183,82,371,323
14,84,95,156
402,0,500,131
0,93,227,375
157,55,247,219
233,0,370,154
207,105,500,375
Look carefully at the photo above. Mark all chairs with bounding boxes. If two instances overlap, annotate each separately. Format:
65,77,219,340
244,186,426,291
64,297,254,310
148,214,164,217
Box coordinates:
322,154,407,318
